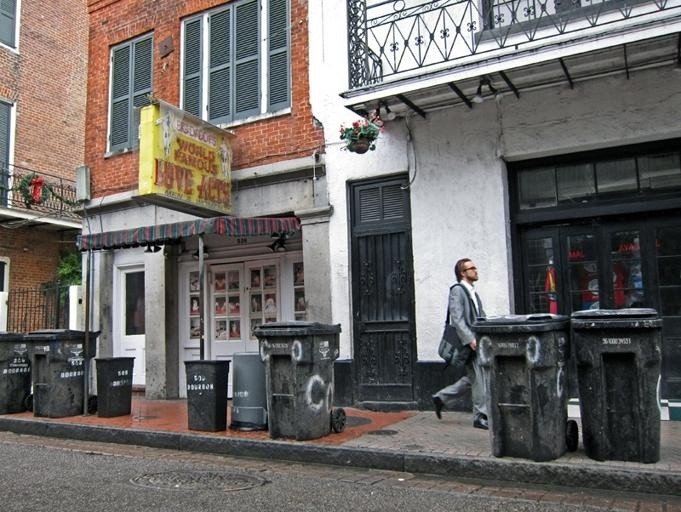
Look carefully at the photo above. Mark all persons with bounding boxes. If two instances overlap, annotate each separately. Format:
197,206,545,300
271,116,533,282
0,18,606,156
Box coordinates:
218,136,231,177
153,111,179,158
428,257,490,432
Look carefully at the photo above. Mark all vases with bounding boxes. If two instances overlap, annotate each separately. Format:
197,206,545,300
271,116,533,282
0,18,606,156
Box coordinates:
351,137,371,154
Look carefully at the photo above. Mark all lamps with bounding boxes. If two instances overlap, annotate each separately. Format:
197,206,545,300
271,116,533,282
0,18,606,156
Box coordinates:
193,245,209,260
266,232,291,253
374,101,397,127
471,79,505,103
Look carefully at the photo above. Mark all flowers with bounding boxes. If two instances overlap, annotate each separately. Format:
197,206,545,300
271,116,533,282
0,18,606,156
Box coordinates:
339,115,386,151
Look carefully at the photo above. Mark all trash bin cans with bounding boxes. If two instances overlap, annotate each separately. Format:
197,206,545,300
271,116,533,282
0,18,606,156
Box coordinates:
0,329,101,418
95,357,136,418
470,308,664,464
229,320,347,441
183,360,231,432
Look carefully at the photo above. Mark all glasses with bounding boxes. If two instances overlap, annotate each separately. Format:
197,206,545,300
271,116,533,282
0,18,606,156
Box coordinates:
463,266,477,272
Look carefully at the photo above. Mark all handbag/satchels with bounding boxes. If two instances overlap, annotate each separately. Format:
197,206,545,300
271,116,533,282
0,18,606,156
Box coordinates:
437,283,475,371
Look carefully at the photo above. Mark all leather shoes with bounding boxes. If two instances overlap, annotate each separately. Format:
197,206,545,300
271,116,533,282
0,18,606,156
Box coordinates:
431,394,444,419
473,415,488,430
